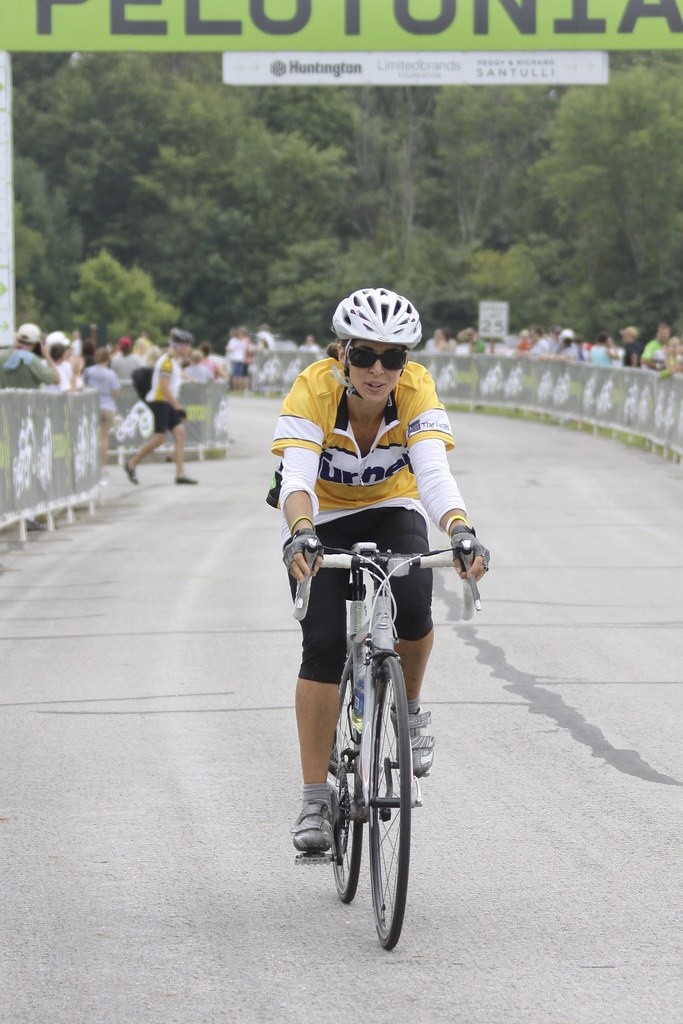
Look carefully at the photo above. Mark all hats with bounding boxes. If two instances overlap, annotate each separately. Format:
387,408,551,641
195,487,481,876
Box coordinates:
119,337,133,348
170,329,194,343
16,323,41,344
620,327,637,338
559,328,574,342
46,332,70,347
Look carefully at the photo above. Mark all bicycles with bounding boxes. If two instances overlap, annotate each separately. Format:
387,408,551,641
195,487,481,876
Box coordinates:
292,535,483,951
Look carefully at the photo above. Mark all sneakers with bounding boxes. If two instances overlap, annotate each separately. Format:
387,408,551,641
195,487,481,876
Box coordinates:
390,707,434,777
291,799,333,850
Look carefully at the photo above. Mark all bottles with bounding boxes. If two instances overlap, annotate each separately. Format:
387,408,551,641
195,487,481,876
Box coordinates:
352,665,367,735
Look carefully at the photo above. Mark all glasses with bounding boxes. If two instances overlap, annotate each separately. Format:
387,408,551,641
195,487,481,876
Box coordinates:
348,346,407,370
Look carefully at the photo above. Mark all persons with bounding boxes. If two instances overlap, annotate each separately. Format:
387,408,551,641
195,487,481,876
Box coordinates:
123,328,197,485
426,322,683,447
83,348,120,488
0,324,320,532
268,288,490,852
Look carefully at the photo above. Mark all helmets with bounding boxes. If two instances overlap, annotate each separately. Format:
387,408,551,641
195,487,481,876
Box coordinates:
333,288,422,349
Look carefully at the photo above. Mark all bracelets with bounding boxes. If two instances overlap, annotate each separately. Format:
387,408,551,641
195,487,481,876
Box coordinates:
290,516,315,536
446,514,468,538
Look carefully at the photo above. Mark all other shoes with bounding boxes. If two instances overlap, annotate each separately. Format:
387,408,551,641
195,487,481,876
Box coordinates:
175,477,197,485
123,462,139,485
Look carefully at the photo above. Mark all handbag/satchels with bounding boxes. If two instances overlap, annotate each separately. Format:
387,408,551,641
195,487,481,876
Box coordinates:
132,367,153,399
